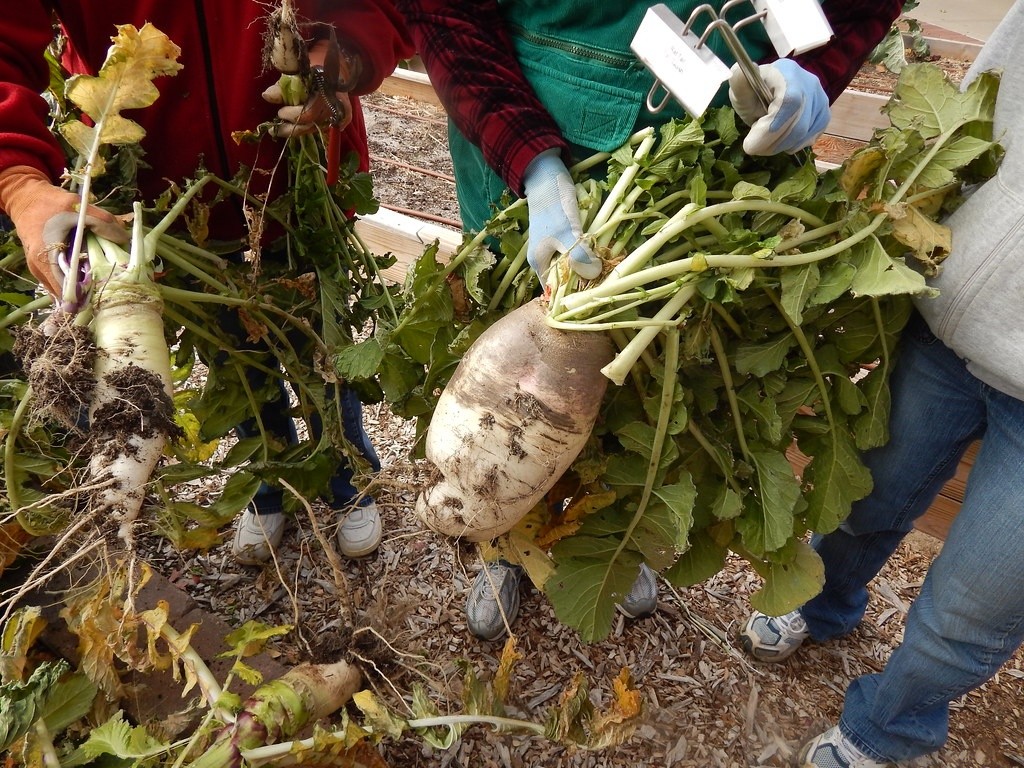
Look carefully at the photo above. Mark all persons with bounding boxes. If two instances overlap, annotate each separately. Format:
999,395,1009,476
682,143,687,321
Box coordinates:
0,0,417,567
416,0,904,640
744,0,1024,768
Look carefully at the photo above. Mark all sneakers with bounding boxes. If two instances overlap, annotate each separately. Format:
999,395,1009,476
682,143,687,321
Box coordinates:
336,500,382,557
466,562,520,642
233,507,287,566
740,608,810,663
798,724,889,768
612,561,659,619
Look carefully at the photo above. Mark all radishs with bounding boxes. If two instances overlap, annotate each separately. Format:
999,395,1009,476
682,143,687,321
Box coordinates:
268,0,303,75
83,303,172,551
416,297,612,542
249,607,364,739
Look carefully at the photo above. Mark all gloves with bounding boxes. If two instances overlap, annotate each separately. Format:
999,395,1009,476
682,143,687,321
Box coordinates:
0,165,131,303
262,36,352,138
522,147,603,291
729,57,832,156
